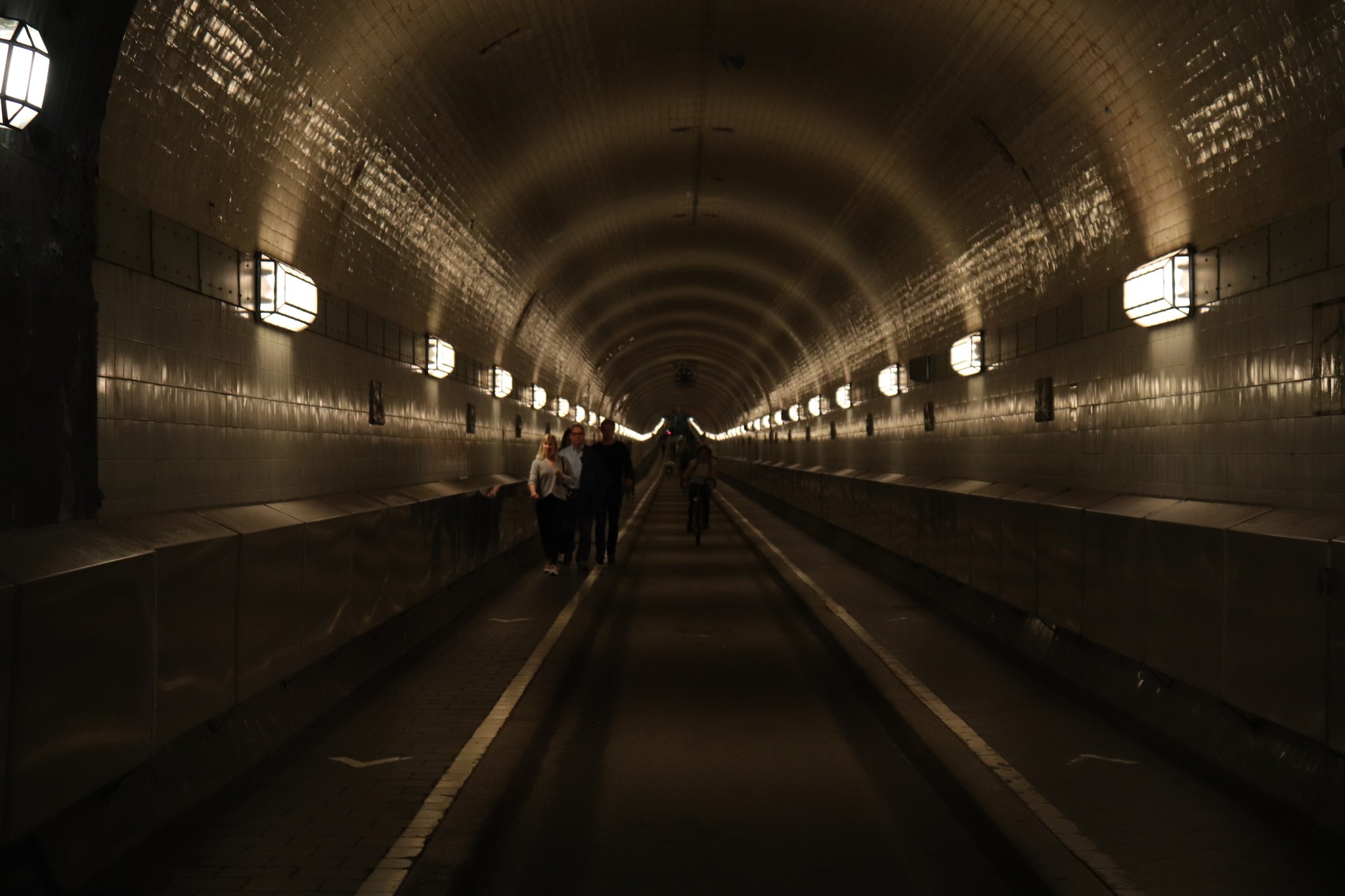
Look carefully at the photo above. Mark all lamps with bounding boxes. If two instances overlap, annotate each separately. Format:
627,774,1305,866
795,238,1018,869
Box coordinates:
835,382,852,409
425,334,455,380
532,383,665,442
492,364,513,398
0,17,52,150
257,251,318,334
807,394,821,418
949,330,984,378
688,403,800,443
876,363,898,398
1122,245,1195,328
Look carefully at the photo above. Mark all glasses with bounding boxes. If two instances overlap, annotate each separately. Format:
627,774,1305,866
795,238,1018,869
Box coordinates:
571,432,583,436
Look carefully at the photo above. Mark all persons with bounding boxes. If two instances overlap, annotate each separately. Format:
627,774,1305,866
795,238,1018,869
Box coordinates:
662,428,719,531
529,434,577,575
557,424,595,571
578,420,634,564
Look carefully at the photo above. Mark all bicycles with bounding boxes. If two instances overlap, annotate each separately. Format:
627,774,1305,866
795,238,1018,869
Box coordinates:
681,479,715,548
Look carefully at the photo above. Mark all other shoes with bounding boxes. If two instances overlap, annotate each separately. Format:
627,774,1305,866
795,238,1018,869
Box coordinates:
595,554,604,564
544,562,560,577
576,558,588,570
687,520,693,531
702,519,710,531
607,552,616,564
563,555,573,564
557,553,565,563
